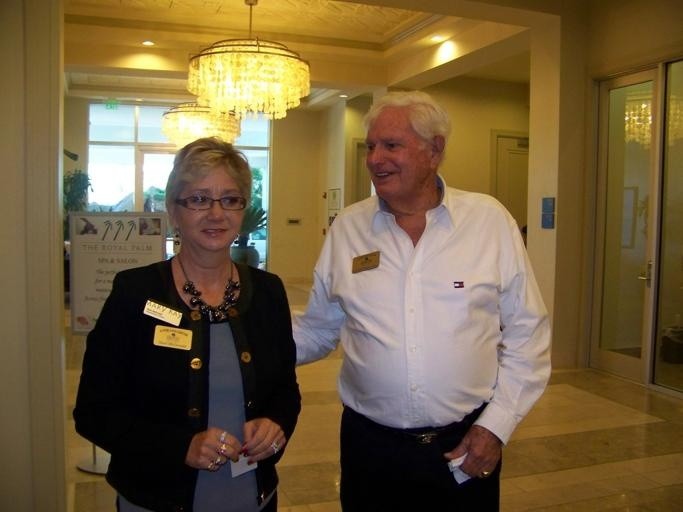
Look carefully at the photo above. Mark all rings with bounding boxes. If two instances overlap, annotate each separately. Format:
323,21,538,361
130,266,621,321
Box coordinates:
480,470,491,478
270,440,281,454
215,443,226,455
211,454,222,465
207,461,214,471
219,431,228,443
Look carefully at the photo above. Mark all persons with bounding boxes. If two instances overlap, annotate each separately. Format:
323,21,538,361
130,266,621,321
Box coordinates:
285,90,553,510
69,133,301,512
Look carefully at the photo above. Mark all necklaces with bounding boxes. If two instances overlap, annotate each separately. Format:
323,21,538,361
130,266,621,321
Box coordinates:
175,250,241,323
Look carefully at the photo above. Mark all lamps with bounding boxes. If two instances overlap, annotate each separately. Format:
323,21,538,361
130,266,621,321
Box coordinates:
162,1,311,147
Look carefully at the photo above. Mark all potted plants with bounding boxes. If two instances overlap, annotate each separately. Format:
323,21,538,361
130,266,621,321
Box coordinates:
231,205,266,269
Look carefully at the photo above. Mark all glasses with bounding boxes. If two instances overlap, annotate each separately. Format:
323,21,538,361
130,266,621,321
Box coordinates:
174,195,248,212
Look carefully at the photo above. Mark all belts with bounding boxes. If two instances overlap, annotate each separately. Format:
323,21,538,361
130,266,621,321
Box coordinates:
347,404,486,449
